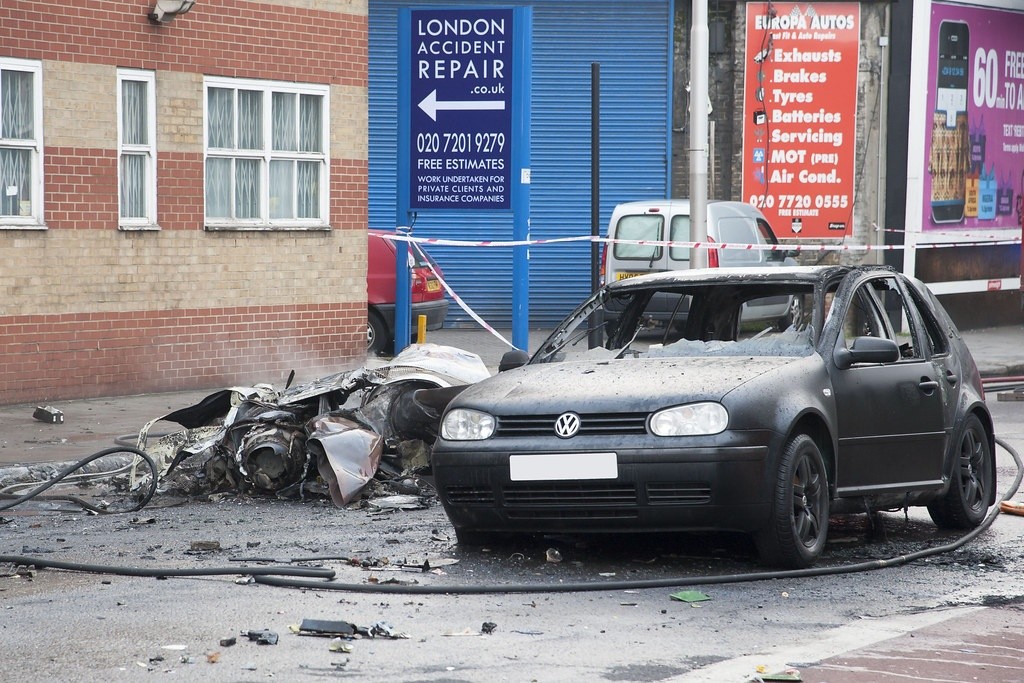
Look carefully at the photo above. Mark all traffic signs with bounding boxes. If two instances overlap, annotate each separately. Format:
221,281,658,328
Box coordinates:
407,10,513,213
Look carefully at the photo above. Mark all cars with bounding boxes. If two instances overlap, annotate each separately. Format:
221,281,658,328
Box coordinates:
428,264,996,572
368,229,448,357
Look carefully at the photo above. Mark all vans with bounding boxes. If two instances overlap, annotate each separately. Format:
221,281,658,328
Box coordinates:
598,201,804,341
